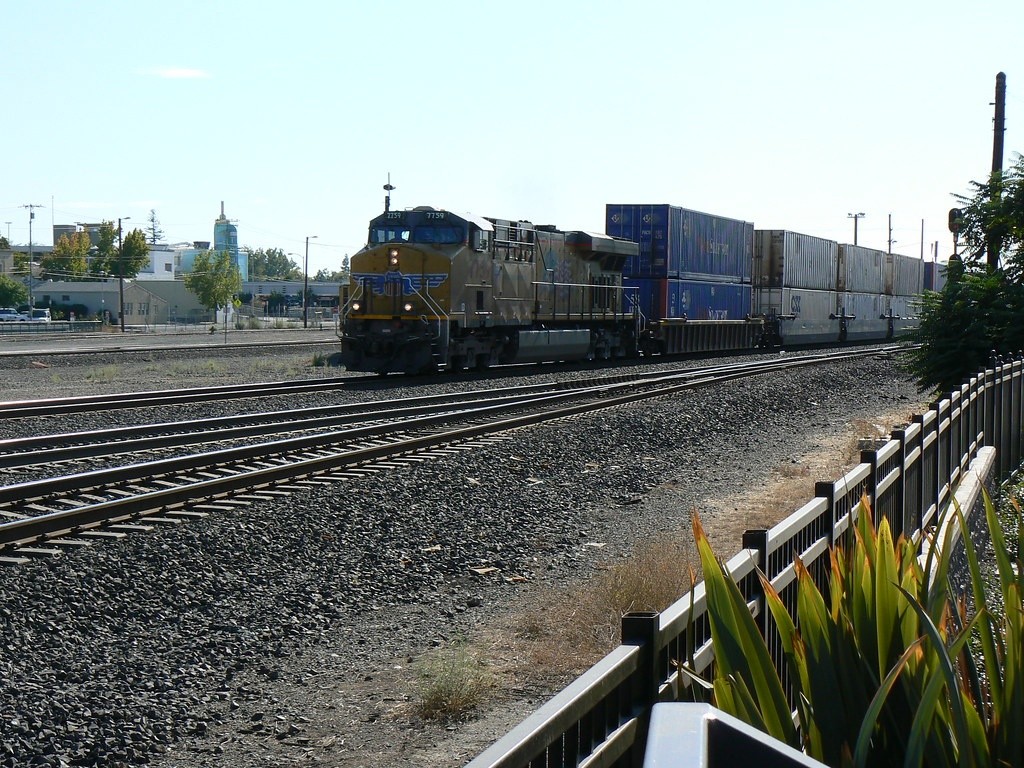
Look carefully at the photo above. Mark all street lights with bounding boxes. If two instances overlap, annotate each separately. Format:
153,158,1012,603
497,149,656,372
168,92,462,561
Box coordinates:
289,253,304,281
304,236,319,328
118,217,131,333
21,203,43,324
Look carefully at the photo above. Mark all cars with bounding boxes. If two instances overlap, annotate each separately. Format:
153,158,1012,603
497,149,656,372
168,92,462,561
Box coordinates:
0,307,52,323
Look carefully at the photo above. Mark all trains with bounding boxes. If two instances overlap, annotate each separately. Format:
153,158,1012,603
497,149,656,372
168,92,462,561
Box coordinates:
335,172,950,378
